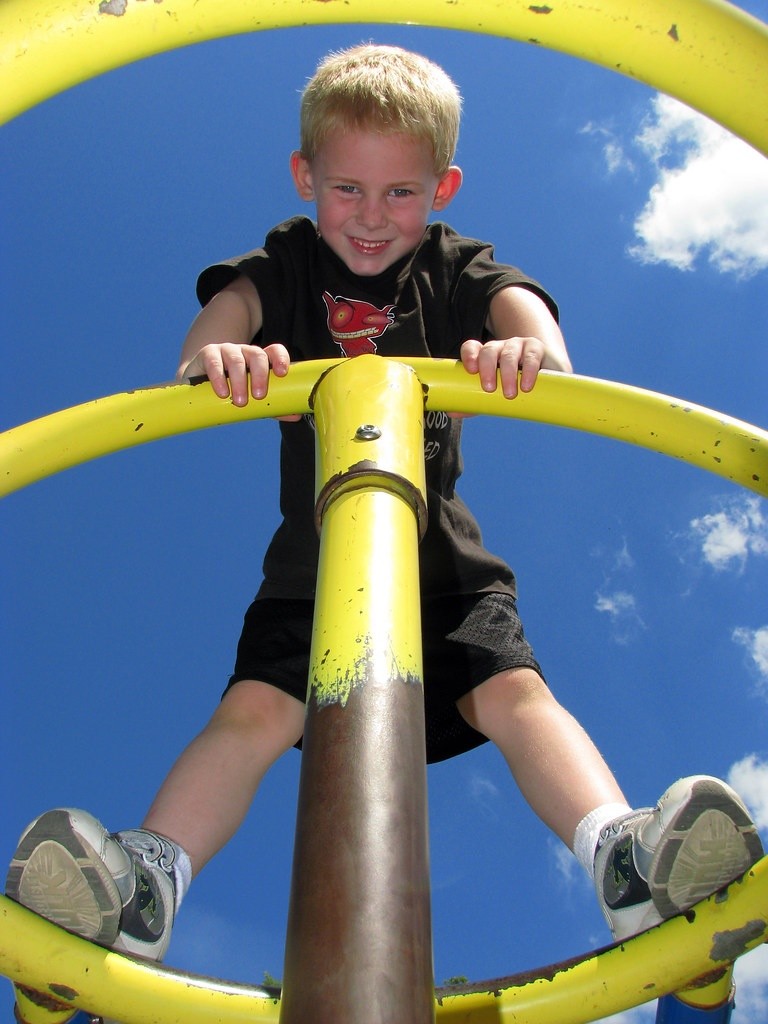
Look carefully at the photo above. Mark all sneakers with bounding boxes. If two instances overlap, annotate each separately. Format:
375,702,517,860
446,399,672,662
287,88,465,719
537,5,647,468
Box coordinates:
593,774,764,943
5,808,180,963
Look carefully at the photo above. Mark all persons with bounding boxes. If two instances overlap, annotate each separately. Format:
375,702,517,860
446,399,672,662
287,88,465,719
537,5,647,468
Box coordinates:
5,41,763,962
